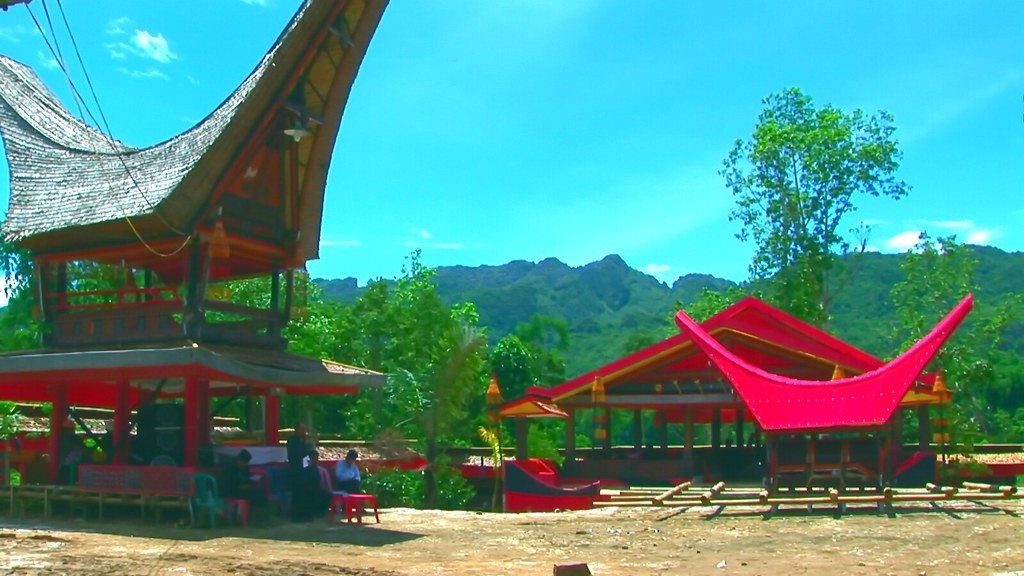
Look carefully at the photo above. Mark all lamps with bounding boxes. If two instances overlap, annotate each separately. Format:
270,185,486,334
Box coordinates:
285,118,309,143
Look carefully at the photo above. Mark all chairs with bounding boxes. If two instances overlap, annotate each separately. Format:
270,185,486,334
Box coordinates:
190,467,335,531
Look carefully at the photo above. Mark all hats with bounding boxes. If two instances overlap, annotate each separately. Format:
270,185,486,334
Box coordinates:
238,450,251,462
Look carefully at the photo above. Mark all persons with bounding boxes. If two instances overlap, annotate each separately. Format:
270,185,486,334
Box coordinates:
333,450,368,514
57,420,91,482
286,422,325,523
218,449,270,528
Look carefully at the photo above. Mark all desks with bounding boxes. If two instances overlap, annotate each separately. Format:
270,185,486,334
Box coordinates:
329,493,378,525
